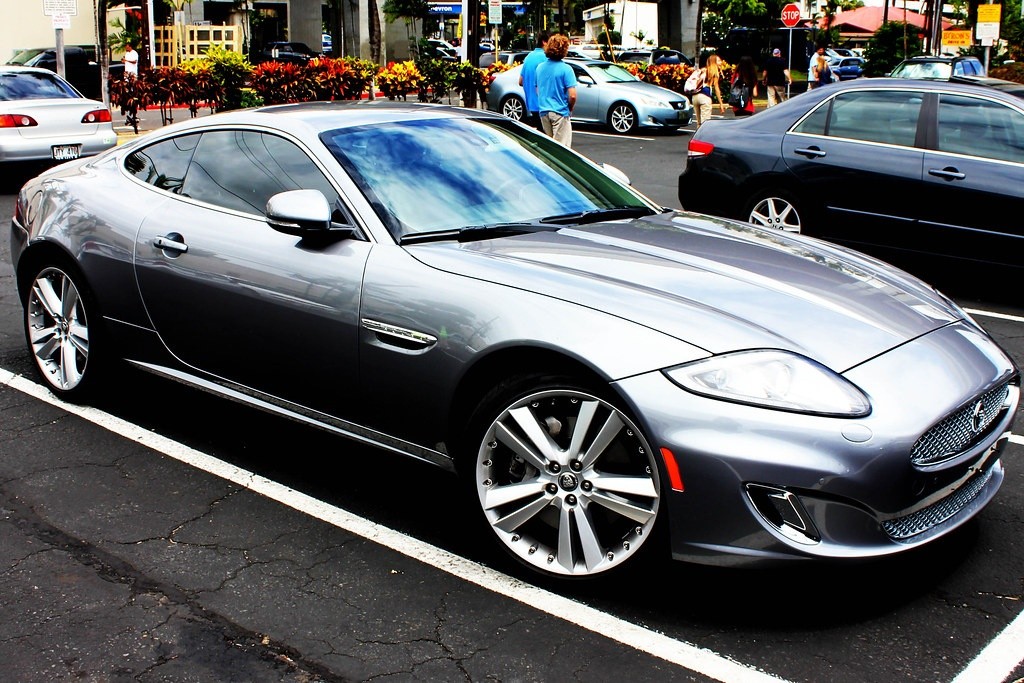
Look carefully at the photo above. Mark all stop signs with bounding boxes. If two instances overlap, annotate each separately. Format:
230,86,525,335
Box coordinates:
780,3,801,28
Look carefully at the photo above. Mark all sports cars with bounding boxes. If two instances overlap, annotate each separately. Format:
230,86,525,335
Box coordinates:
6,98,1023,599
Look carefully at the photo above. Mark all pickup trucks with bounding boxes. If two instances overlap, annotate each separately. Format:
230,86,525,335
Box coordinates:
6,44,124,98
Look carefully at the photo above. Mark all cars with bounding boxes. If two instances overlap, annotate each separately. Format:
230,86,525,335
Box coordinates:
835,74,1024,152
483,57,694,136
422,33,462,60
322,33,333,53
0,63,118,162
823,46,870,79
617,46,693,68
479,41,591,68
258,41,322,67
675,75,1024,276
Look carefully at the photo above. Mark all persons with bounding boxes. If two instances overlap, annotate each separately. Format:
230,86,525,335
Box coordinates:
731,55,758,116
452,39,461,64
121,43,138,126
762,48,791,108
807,46,832,91
692,55,725,128
516,28,526,50
519,30,577,149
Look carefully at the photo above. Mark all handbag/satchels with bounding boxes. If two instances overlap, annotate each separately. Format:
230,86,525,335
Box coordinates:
684,69,706,94
728,77,749,108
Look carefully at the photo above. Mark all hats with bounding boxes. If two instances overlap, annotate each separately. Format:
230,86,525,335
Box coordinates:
773,48,780,53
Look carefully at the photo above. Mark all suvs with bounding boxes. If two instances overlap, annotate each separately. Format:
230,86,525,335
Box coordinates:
885,51,986,82
